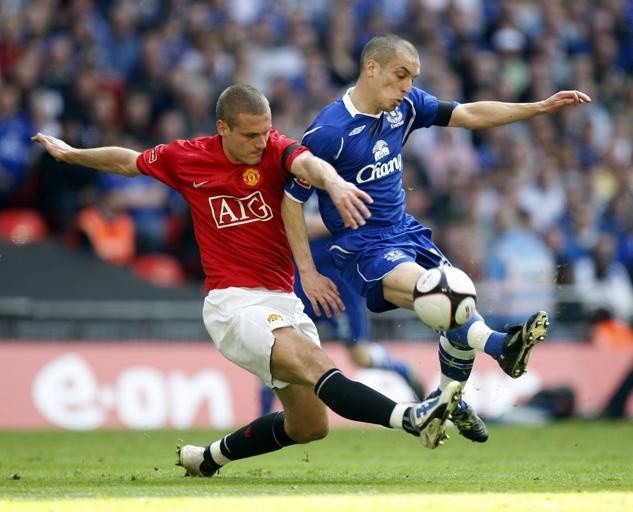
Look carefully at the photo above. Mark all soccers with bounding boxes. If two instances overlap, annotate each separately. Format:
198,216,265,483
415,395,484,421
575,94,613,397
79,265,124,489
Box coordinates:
413,267,476,332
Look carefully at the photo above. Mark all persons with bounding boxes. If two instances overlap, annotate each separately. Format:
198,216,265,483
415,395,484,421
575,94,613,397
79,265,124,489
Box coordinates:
280,34,592,441
32,83,464,477
0,1,633,428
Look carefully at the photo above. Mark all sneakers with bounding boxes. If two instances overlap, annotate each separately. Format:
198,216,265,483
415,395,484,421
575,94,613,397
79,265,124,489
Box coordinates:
174,443,215,477
494,310,550,380
415,380,489,449
